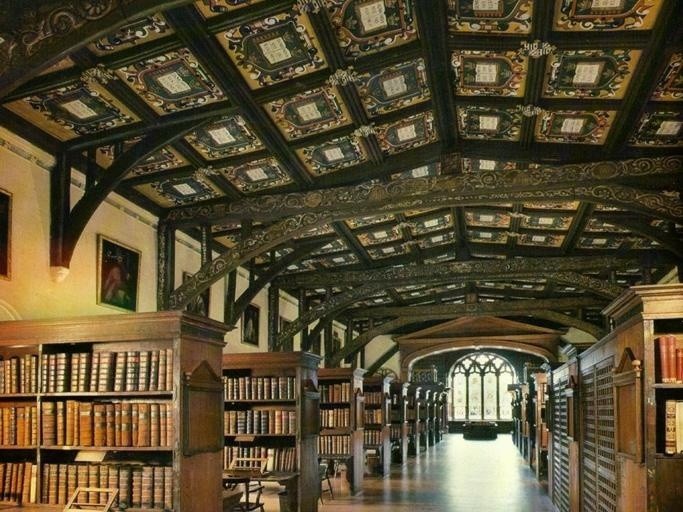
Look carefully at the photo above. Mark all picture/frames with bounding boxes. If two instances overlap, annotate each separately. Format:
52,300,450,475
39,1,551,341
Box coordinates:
0,188,14,282
184,271,212,316
96,233,142,313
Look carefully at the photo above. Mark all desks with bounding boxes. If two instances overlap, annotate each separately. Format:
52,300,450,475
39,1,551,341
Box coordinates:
463,423,498,439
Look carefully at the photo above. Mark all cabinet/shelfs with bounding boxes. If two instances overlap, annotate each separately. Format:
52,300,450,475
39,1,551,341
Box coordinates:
507,284,683,512
0,302,450,512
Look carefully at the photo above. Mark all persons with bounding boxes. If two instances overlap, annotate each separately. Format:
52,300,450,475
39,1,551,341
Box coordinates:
103,255,130,301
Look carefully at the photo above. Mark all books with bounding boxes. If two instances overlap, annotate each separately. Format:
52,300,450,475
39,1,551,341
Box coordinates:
391,393,400,439
41,349,173,510
407,395,415,434
541,383,548,448
363,391,383,445
0,349,37,504
318,382,351,455
224,376,296,472
419,398,425,433
654,334,683,457
